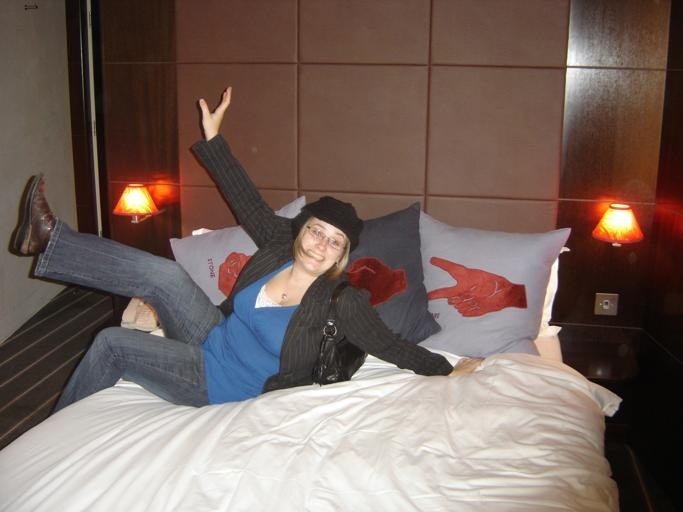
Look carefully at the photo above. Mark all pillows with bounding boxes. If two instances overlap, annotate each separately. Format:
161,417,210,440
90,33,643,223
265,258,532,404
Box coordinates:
342,200,443,345
173,197,573,360
169,198,307,306
418,211,573,359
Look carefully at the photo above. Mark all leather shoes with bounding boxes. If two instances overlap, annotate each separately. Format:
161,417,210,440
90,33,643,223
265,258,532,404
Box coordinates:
13,172,58,256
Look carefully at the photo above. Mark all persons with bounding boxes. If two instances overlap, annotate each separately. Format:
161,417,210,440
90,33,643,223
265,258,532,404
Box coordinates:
9,86,482,422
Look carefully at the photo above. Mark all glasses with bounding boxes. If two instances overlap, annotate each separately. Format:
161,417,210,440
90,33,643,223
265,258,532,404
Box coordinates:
305,225,347,251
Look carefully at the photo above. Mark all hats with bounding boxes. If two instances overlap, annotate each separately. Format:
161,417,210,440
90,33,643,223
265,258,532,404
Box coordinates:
291,195,365,253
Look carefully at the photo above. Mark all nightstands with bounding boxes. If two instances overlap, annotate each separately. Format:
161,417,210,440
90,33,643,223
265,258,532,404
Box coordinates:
561,325,648,433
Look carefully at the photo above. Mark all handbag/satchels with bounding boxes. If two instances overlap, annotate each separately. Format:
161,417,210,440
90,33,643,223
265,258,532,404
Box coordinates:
312,331,366,386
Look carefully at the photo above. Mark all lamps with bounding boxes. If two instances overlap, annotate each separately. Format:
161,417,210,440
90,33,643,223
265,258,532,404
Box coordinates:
112,181,165,225
590,202,644,248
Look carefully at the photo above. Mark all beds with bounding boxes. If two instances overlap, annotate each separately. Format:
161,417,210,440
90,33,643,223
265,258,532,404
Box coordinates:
0,199,624,511
